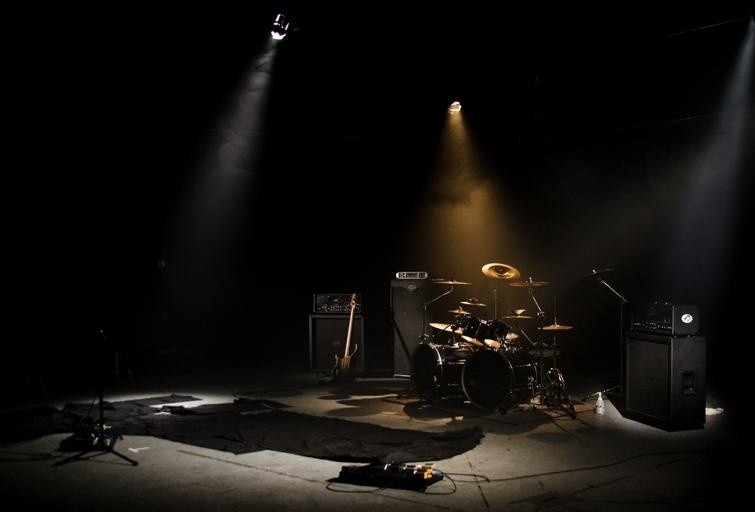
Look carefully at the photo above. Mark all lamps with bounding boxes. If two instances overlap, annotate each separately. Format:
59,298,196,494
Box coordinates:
271,10,289,41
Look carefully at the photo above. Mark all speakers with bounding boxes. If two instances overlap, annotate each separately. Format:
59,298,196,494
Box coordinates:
390,281,433,378
309,314,365,372
622,333,708,432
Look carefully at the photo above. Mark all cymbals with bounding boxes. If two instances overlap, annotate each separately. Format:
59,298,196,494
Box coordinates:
460,301,486,307
448,310,472,314
509,281,551,287
503,315,533,318
433,281,471,284
482,263,520,280
538,325,573,330
430,323,462,334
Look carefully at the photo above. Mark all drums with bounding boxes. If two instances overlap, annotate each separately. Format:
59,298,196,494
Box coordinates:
505,322,521,340
461,346,540,409
484,320,509,348
410,343,475,407
580,269,615,278
461,315,490,346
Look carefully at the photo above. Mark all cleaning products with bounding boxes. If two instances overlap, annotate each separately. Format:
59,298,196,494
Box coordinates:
596,391,606,416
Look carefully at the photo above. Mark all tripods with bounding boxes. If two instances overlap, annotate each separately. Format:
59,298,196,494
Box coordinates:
499,291,576,420
54,387,138,466
582,279,628,402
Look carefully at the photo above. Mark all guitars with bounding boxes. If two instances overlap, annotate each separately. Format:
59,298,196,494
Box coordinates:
331,292,358,381
